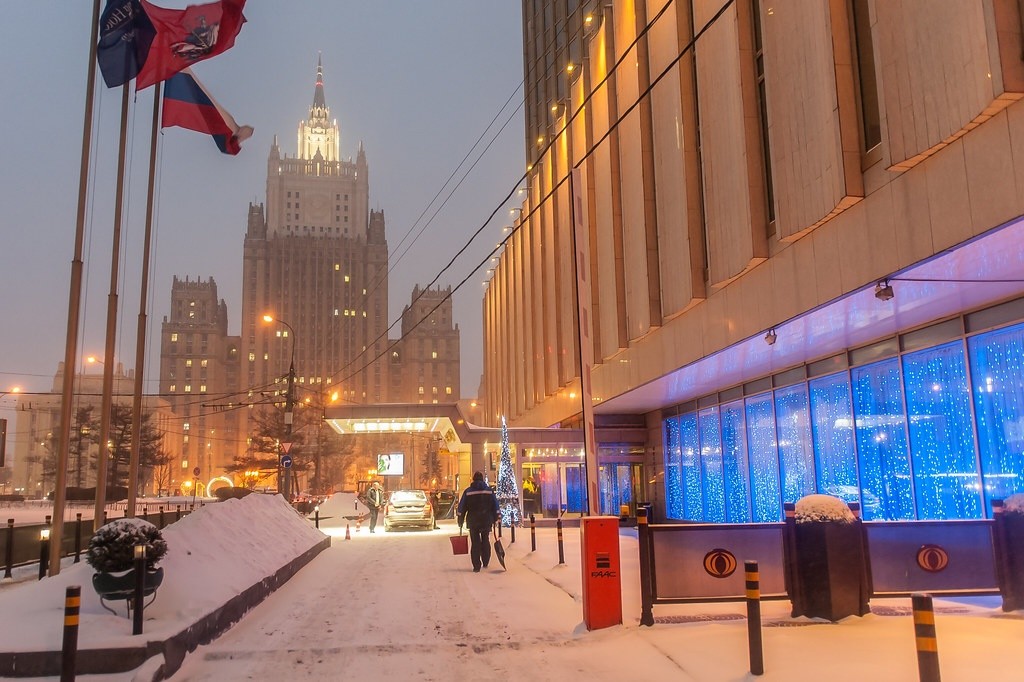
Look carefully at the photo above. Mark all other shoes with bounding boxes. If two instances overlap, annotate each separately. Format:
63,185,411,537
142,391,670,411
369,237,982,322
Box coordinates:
473,567,480,572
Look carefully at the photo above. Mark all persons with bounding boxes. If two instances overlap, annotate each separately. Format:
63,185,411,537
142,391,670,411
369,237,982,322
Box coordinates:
367,482,384,533
377,454,394,474
457,472,502,573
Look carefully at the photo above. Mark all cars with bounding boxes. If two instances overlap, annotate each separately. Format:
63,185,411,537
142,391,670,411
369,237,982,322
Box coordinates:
381,489,457,532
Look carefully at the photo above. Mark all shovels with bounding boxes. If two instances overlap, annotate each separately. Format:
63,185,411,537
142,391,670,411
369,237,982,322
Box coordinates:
492,523,507,572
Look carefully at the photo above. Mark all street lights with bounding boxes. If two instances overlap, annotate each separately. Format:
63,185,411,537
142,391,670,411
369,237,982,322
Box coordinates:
261,312,295,502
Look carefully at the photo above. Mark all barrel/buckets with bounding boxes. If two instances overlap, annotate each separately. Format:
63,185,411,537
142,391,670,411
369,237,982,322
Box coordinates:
450,526,468,555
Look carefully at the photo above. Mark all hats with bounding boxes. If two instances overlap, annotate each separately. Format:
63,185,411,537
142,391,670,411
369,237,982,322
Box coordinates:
473,472,483,481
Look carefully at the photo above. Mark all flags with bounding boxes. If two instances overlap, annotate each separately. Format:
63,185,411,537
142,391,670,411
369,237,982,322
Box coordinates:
98,0,255,156
